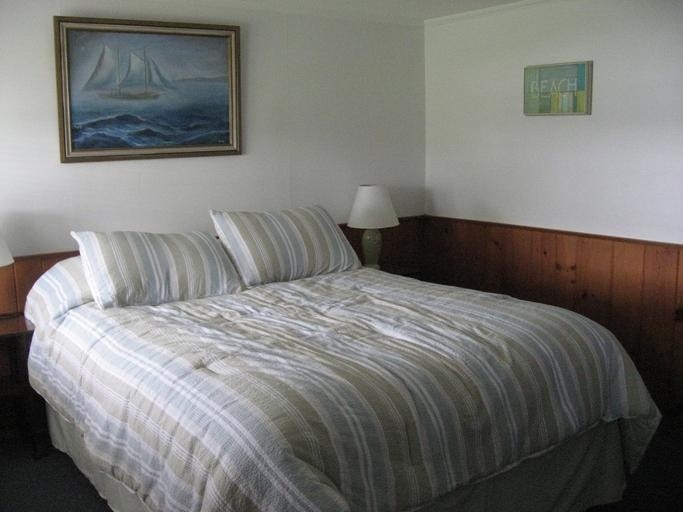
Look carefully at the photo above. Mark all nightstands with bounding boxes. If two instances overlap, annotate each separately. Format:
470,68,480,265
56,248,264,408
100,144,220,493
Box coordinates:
0,315,46,460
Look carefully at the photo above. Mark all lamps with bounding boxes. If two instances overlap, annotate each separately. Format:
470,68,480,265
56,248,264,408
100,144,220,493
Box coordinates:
346,183,401,270
0,237,15,267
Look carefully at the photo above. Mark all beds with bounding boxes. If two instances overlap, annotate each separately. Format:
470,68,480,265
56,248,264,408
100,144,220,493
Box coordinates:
12,250,662,511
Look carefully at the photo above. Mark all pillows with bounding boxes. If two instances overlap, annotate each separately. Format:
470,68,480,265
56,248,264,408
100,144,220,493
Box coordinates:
71,230,244,312
209,203,362,290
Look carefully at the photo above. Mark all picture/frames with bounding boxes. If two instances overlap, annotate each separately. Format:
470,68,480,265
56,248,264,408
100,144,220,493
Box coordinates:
523,60,591,116
52,15,242,164
